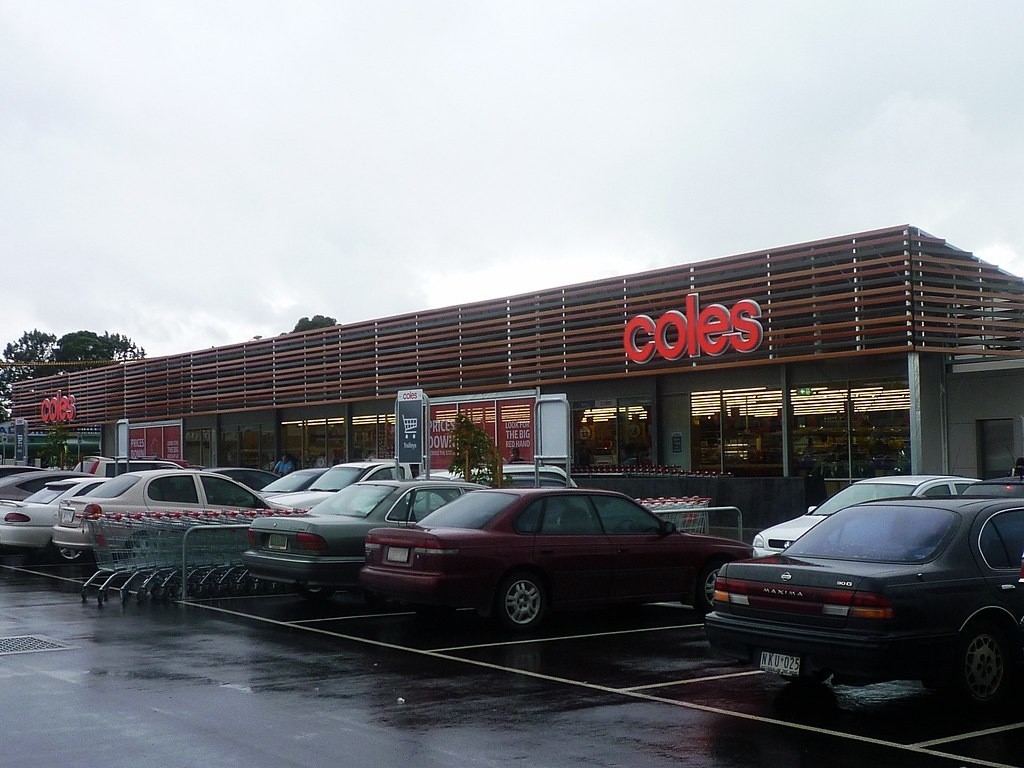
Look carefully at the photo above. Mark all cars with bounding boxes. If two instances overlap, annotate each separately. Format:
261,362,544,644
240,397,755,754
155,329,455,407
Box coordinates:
0,455,283,569
702,477,1024,726
243,462,590,614
358,488,751,640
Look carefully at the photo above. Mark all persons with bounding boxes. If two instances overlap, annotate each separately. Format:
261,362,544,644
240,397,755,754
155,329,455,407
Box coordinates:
620,445,637,465
746,446,761,458
1008,457,1024,476
272,454,295,475
508,447,524,463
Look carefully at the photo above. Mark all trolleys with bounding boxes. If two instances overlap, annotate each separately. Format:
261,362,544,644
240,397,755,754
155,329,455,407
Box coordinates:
75,511,310,611
565,464,732,526
599,497,712,535
